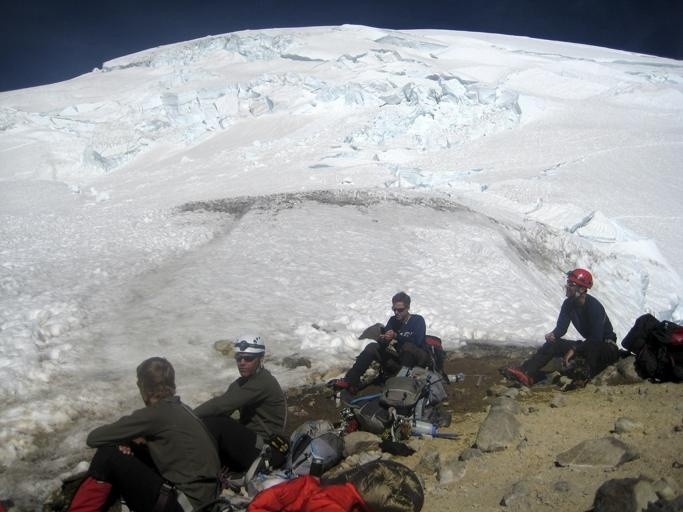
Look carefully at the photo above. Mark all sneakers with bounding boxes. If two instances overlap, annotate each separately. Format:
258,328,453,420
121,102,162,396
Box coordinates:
498,364,534,389
325,374,361,395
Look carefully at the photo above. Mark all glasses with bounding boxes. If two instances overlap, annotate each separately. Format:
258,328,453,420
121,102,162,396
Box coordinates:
567,279,577,287
233,353,260,362
391,305,408,312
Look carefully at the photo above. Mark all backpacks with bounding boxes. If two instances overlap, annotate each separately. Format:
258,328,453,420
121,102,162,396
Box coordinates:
286,413,347,477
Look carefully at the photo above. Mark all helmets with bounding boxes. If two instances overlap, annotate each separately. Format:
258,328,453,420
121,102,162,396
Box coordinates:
567,268,594,289
231,334,266,357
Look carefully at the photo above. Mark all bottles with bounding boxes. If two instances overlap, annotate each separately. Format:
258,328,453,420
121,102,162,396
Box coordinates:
405,418,432,436
440,370,466,384
308,455,324,479
334,392,341,408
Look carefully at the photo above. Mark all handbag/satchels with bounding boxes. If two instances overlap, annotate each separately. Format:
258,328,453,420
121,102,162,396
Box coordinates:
348,360,452,433
417,332,447,371
620,310,683,386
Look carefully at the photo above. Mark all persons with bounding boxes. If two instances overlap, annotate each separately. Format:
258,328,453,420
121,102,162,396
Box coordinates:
499,268,619,391
193,334,288,474
66,357,224,512
333,291,433,395
